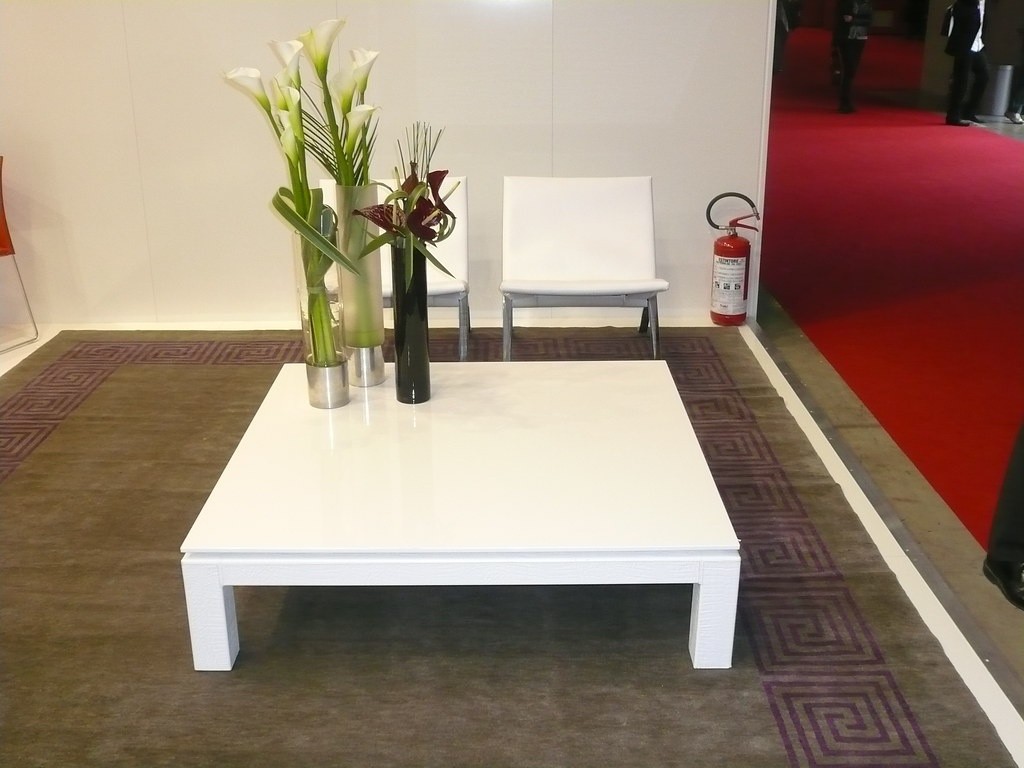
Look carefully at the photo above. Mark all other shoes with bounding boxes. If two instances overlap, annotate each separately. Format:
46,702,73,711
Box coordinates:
1004,109,1024,124
964,114,987,123
946,119,970,127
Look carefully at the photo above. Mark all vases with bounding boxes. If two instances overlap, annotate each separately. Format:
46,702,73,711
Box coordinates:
393,238,431,403
294,230,349,410
335,175,386,387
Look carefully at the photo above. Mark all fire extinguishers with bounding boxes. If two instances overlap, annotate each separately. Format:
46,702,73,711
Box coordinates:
706,192,760,325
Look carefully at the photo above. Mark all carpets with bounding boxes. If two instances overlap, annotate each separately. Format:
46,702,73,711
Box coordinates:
0,326,1016,767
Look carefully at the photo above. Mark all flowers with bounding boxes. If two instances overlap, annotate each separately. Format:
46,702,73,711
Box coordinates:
353,121,459,278
224,39,333,239
280,21,384,185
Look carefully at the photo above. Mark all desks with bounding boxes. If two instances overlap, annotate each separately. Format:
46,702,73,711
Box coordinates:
181,359,740,671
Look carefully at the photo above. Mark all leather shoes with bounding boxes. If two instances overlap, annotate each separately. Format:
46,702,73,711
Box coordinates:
982,556,1024,610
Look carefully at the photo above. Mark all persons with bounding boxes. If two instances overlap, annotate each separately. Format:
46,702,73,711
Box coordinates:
984,423,1024,611
1005,64,1024,124
772,0,795,68
831,0,872,113
944,0,991,126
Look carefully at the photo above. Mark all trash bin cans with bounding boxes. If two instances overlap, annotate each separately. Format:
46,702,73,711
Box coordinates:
979,64,1015,116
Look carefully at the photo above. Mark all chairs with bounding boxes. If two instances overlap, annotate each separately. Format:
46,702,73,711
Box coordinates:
318,178,472,361
0,157,39,355
499,174,669,359
